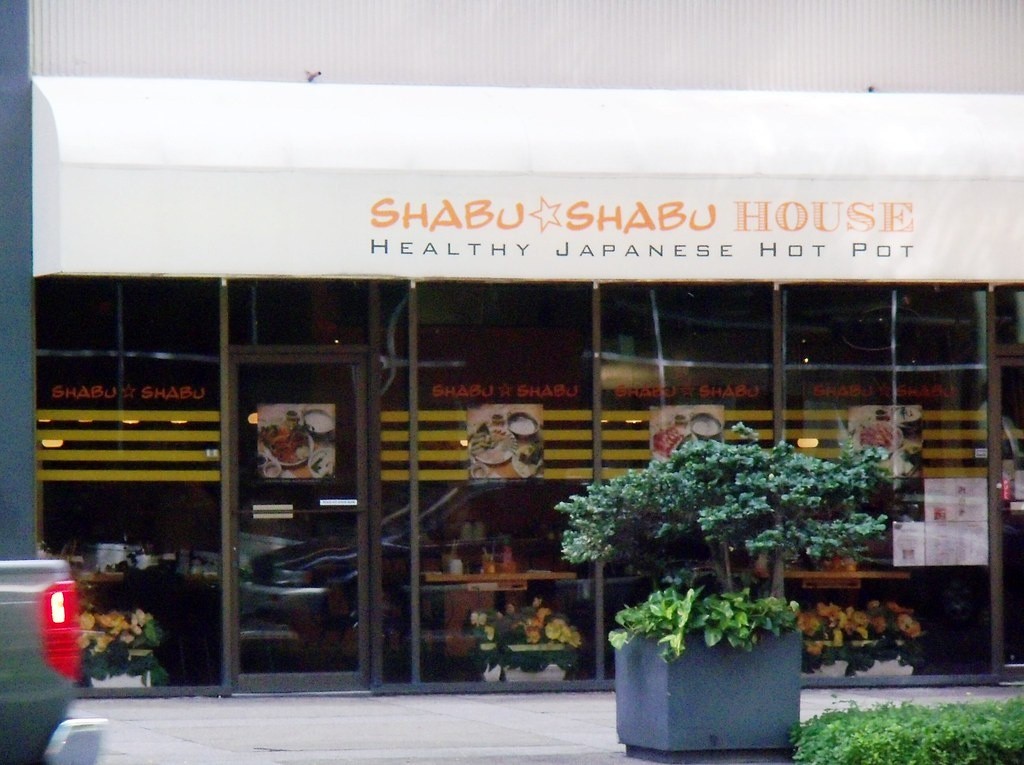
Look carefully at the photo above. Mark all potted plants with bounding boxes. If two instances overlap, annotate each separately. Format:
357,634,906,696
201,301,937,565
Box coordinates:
554,422,902,764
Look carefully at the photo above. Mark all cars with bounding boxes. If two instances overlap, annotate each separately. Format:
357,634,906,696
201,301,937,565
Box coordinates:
237,540,398,667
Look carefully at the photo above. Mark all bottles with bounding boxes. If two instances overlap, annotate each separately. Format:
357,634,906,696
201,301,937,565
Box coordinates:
502,536,513,563
449,559,463,575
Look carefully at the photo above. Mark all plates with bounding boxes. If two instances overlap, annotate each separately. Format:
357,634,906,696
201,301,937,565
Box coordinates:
258,408,336,478
468,410,543,480
852,406,922,458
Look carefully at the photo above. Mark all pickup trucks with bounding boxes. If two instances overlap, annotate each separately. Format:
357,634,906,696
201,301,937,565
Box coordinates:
0,554,109,765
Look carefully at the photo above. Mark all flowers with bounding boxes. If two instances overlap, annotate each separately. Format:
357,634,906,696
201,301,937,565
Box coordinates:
79,608,167,683
798,599,924,678
469,597,581,671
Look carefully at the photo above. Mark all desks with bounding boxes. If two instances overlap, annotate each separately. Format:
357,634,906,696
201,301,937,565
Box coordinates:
414,571,576,660
723,567,909,600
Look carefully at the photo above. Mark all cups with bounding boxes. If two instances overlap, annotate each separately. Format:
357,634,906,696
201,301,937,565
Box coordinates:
481,554,496,573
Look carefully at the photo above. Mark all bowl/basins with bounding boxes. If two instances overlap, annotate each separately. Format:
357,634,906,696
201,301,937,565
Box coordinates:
273,567,312,587
507,412,540,439
691,413,722,441
303,409,335,439
892,406,922,434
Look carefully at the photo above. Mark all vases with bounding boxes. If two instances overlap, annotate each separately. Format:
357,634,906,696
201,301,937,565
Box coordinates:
89,649,151,688
799,637,849,678
850,640,916,678
478,643,577,681
